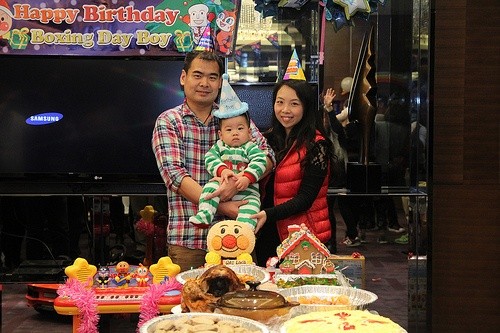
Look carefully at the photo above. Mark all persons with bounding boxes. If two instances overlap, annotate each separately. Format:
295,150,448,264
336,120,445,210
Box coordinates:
317,85,408,247
189,74,267,231
250,47,335,251
409,109,427,233
150,22,277,273
115,261,131,289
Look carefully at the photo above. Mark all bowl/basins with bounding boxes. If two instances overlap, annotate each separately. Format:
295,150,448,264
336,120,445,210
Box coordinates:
174,264,271,287
209,282,299,319
139,312,269,333
276,285,378,311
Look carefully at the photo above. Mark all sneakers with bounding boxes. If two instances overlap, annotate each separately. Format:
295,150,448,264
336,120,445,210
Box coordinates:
376,233,388,244
365,223,378,232
388,224,405,233
339,236,363,247
395,233,408,244
356,231,367,243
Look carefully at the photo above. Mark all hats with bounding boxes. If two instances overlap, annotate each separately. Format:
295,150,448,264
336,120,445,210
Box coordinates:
212,73,249,119
193,22,215,52
283,48,306,81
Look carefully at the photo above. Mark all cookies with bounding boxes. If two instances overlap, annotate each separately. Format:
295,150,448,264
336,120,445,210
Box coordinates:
153,316,259,333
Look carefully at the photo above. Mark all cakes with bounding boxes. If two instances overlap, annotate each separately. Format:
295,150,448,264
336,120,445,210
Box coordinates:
284,310,406,333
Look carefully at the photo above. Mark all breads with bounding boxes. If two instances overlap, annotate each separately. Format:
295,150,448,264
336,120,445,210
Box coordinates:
284,295,350,305
